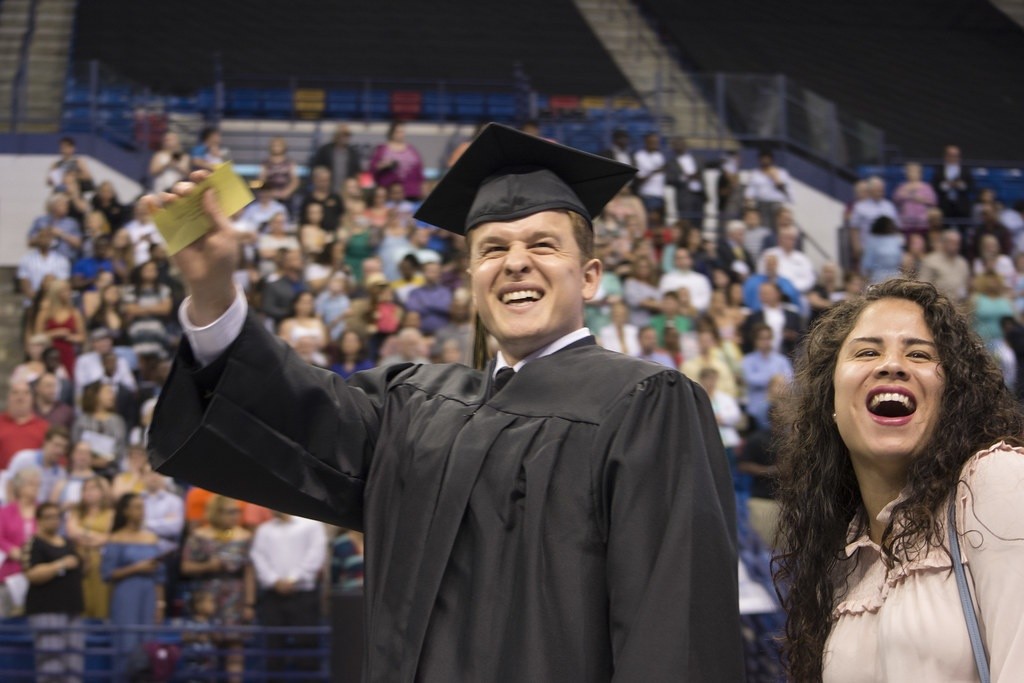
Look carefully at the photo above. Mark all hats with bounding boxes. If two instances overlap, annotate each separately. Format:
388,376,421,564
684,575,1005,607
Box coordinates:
414,123,639,238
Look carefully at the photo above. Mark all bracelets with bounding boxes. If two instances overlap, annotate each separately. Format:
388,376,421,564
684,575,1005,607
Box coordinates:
57,558,66,576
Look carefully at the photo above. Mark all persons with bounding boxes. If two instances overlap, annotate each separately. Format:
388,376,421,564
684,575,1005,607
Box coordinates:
331,528,366,681
179,493,252,682
20,499,88,683
765,276,1024,683
1,118,1024,617
103,489,166,683
139,123,747,683
252,505,329,682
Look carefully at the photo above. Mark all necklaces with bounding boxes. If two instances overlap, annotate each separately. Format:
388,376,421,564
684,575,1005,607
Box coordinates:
210,523,236,543
